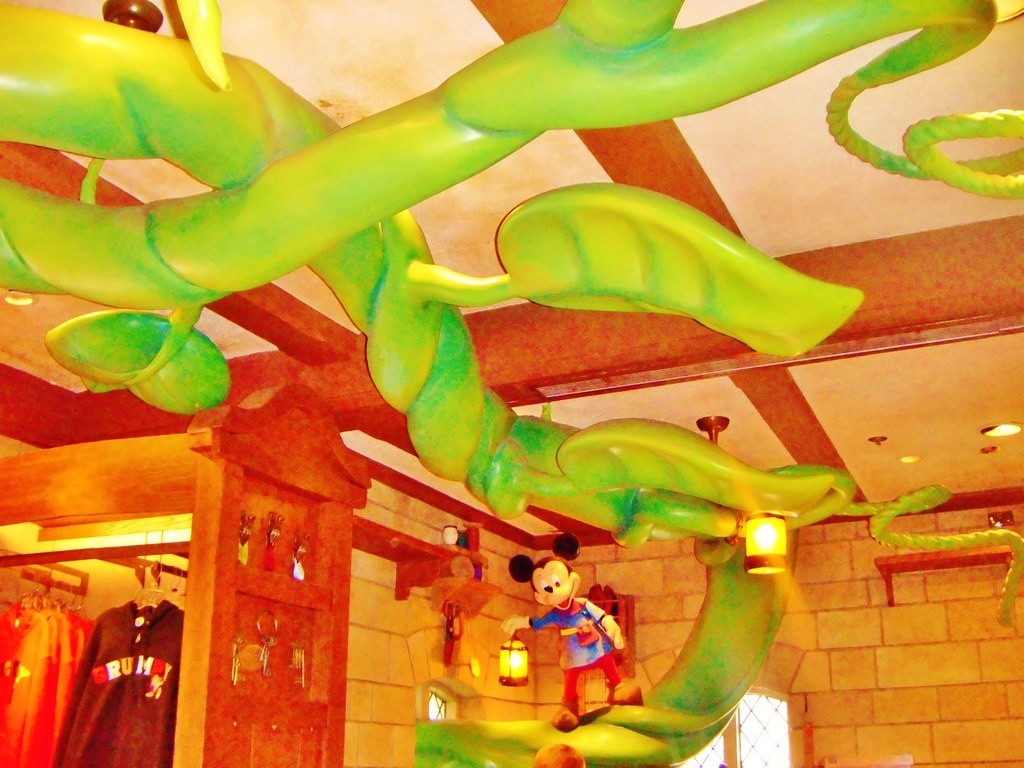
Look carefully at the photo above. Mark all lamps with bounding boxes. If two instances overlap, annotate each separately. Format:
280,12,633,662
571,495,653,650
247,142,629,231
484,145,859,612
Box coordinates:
498,633,530,688
